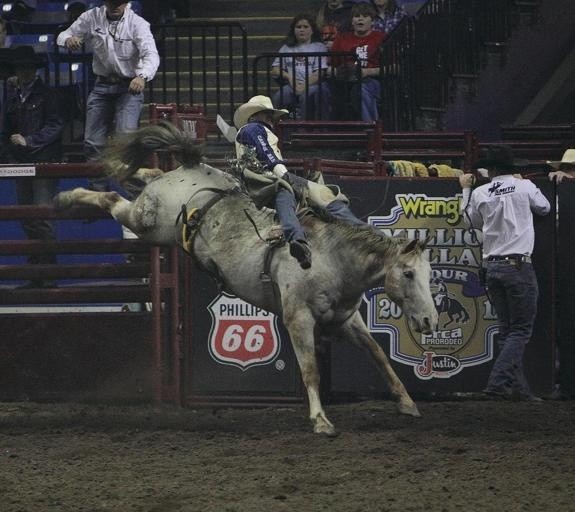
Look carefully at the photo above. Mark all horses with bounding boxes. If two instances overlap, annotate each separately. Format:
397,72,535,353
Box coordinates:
52,121,439,437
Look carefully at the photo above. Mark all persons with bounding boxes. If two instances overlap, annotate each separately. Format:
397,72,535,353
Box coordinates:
232,93,391,270
54,1,161,191
1,45,64,290
268,0,407,123
532,148,574,401
458,149,552,402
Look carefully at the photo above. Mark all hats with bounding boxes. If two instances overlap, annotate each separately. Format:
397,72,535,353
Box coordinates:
6,45,48,69
546,149,575,169
233,95,289,129
472,143,529,168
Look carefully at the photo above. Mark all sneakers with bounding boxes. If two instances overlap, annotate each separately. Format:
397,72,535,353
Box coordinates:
289,240,312,269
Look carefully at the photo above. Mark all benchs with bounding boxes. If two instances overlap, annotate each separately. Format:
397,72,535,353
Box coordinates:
0,0,142,140
